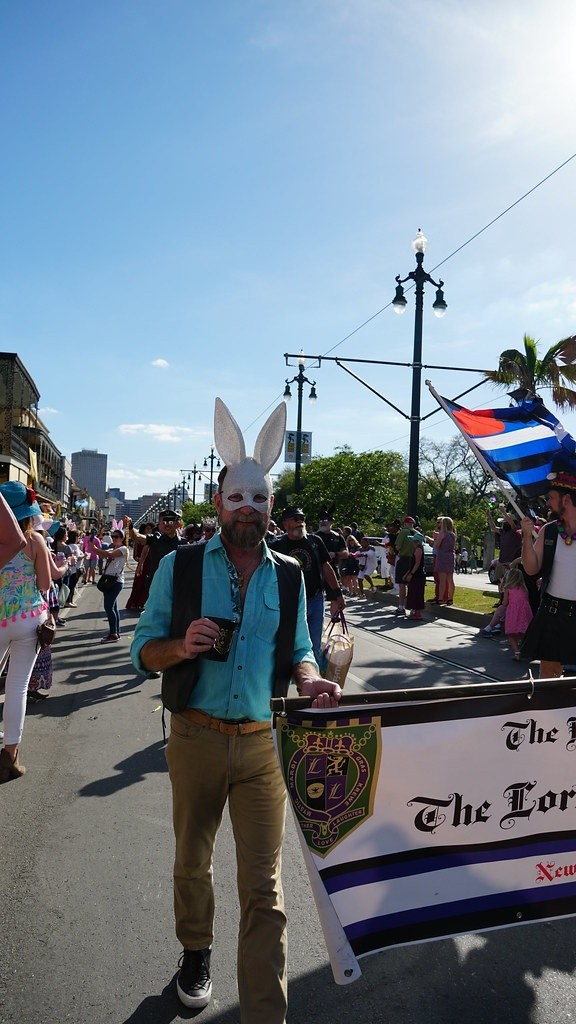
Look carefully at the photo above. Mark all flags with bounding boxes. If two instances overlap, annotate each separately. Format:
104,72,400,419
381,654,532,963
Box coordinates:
440,395,576,499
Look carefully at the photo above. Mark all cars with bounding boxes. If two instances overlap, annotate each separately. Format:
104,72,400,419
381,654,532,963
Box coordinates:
488,557,504,584
421,543,439,574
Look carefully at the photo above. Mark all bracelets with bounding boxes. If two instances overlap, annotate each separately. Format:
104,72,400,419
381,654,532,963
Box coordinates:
411,573,413,575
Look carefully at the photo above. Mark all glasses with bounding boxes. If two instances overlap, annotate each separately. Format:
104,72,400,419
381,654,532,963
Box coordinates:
162,520,174,525
206,527,216,532
437,521,440,524
286,516,304,521
319,520,329,523
111,535,120,539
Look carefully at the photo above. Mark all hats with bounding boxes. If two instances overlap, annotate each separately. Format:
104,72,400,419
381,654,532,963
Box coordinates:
282,506,305,519
0,480,60,536
160,509,181,521
545,471,576,493
349,522,358,529
402,516,416,524
407,534,423,541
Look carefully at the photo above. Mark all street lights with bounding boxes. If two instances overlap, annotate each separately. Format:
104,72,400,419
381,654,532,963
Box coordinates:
178,475,190,505
204,442,221,504
134,482,182,529
389,230,447,528
282,349,318,504
187,463,202,505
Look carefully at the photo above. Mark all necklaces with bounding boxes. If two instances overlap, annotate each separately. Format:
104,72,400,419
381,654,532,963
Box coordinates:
126,517,134,572
555,521,576,545
235,562,243,587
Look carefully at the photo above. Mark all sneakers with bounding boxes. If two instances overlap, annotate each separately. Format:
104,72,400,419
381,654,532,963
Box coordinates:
177,945,212,1008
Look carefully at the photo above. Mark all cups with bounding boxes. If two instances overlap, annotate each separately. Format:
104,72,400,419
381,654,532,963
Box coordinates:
200,615,239,662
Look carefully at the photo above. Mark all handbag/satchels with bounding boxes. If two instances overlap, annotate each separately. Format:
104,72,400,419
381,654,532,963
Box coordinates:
97,575,117,592
323,610,353,689
36,614,56,650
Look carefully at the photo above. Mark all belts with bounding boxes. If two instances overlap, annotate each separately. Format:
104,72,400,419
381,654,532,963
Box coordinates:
178,709,271,735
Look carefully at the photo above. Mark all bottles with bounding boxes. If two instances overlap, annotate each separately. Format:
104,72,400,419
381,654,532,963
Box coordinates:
57,552,65,561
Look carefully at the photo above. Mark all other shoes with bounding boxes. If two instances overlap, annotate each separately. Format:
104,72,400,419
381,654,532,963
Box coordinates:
512,650,521,660
53,577,121,643
475,628,493,637
27,691,49,699
341,587,453,620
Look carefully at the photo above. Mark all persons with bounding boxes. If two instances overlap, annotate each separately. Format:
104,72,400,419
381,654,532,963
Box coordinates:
486,507,522,564
515,513,547,547
0,481,131,781
124,457,342,1024
520,472,576,679
455,548,479,574
502,569,532,660
271,507,346,696
475,565,540,637
313,511,377,623
375,516,426,619
426,516,456,604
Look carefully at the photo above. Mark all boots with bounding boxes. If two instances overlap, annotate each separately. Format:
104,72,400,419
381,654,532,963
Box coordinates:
0,747,25,779
